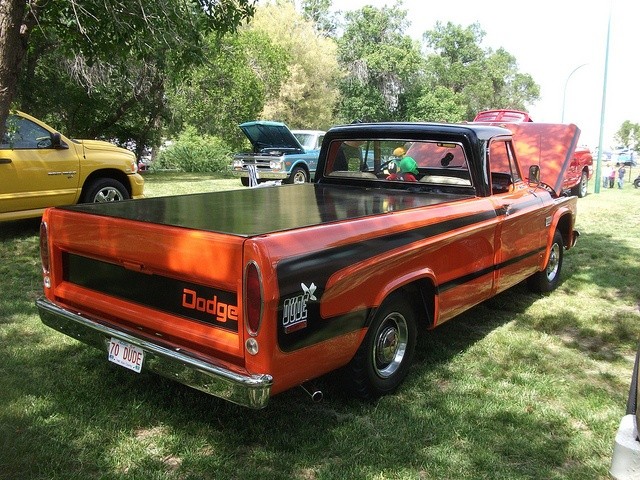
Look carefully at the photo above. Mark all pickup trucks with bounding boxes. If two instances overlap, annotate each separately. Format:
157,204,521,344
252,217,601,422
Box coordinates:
232,121,325,186
36,123,580,410
475,110,594,198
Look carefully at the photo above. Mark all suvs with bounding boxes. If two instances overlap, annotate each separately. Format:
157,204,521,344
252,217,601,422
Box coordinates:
0,110,147,221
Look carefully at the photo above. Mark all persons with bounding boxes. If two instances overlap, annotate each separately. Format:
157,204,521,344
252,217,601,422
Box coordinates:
602,164,625,189
335,119,368,171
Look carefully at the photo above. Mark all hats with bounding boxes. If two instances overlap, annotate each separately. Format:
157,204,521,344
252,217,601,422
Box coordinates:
394,148,405,156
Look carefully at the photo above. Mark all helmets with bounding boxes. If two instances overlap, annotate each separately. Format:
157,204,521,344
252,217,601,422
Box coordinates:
399,157,419,175
